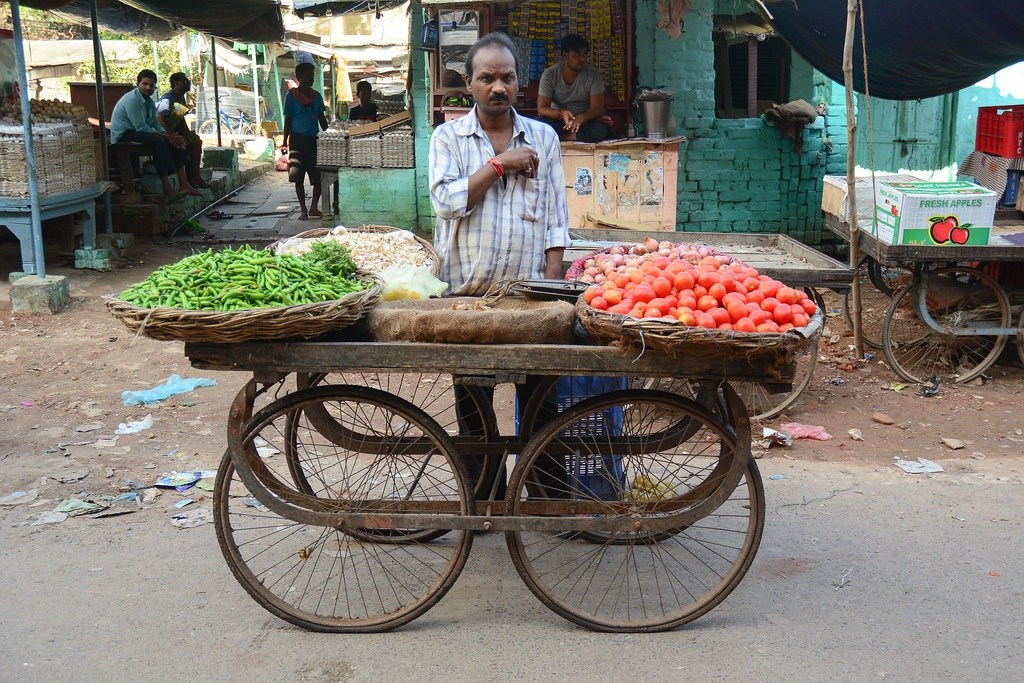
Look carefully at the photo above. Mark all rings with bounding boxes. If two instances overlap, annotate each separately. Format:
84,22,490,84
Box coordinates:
524,167,532,172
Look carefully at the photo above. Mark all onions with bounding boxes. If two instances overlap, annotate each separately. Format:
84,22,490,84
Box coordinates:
580,236,741,282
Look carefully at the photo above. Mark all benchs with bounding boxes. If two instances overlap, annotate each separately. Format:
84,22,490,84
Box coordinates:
107,141,149,192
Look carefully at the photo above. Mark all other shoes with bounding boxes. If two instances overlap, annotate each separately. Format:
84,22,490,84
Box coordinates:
189,178,211,188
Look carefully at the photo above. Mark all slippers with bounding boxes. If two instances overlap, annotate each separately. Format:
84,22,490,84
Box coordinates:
206,211,224,217
210,213,234,219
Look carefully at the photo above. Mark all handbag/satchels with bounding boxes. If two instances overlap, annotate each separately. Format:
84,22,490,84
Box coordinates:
277,155,289,172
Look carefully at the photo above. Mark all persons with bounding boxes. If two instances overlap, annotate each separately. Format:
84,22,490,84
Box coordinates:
110,69,203,205
428,31,581,541
281,62,328,220
533,34,614,142
349,81,379,122
153,72,212,189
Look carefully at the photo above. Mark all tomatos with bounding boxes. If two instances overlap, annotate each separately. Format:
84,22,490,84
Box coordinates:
583,257,816,333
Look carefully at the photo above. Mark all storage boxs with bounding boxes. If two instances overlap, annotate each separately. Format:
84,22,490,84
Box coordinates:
976,104,1024,160
974,151,1024,208
514,375,629,516
348,107,412,138
820,173,926,224
869,180,997,247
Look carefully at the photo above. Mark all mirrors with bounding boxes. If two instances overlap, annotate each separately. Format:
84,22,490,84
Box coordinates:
438,8,480,90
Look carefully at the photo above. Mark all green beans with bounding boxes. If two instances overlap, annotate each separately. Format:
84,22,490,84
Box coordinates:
116,244,374,311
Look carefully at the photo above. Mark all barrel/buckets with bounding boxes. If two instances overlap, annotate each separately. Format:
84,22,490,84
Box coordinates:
637,98,674,141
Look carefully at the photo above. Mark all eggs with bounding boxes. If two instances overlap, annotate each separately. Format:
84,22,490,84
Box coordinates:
318,100,411,139
0,98,88,137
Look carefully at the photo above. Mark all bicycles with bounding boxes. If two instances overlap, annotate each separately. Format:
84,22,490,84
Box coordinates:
198,108,268,138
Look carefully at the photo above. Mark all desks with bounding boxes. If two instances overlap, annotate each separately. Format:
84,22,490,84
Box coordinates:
0,183,106,274
315,166,338,216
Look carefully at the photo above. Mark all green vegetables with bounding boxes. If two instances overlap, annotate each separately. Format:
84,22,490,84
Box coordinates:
299,235,359,278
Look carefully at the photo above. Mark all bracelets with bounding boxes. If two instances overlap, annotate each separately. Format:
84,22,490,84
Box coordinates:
487,157,505,176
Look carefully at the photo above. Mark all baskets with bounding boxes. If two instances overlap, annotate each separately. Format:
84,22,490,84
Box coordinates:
268,224,441,280
575,289,825,359
102,264,386,345
515,369,627,503
567,243,648,281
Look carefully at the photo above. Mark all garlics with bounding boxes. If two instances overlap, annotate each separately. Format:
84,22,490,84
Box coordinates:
333,226,347,235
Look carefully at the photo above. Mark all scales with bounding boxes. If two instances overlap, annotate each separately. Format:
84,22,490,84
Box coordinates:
506,279,589,303
